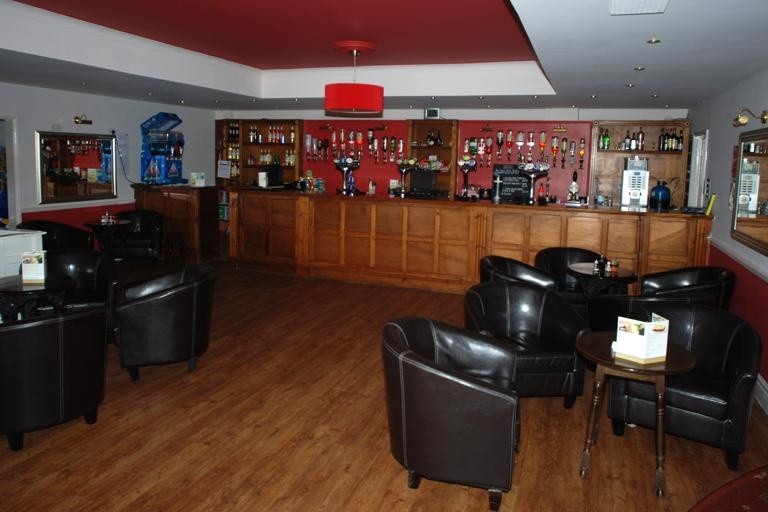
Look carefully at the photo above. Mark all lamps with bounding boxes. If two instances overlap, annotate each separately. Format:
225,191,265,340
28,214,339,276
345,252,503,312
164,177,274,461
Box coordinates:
322,39,385,115
74,114,92,125
729,106,767,128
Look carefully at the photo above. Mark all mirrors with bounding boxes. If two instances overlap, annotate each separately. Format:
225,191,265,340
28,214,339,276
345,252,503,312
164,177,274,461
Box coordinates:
729,127,767,257
34,129,118,205
0,113,22,236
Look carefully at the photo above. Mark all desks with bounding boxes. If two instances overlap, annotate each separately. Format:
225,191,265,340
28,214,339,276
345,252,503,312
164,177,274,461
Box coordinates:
0,271,80,299
573,328,696,500
565,259,642,301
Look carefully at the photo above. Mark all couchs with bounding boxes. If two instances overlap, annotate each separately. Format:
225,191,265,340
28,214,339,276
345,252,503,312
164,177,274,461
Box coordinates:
15,208,172,294
1,290,110,449
113,262,218,383
374,314,526,512
475,252,558,290
460,281,591,413
532,245,631,329
606,302,763,471
639,265,737,312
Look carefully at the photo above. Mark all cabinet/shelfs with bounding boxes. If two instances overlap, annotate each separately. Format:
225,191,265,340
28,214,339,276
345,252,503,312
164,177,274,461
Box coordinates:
0,228,48,279
217,120,297,187
589,119,690,210
406,121,456,199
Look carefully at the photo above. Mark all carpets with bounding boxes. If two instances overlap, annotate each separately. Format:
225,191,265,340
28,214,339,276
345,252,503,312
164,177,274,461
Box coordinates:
686,463,767,512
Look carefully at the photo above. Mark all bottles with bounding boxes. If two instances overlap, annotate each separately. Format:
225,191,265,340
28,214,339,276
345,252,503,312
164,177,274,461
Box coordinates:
551,136,559,157
594,254,619,273
561,138,567,158
226,142,239,159
426,129,443,146
539,131,548,154
620,126,644,150
306,127,404,163
246,146,296,167
249,123,295,144
506,130,513,155
570,141,576,157
527,132,535,152
228,123,238,139
598,128,603,149
368,176,376,192
231,162,240,178
567,171,580,202
515,132,525,152
496,129,503,154
302,177,324,193
538,183,544,203
580,139,585,167
465,137,492,168
603,128,610,149
658,125,685,152
100,209,119,225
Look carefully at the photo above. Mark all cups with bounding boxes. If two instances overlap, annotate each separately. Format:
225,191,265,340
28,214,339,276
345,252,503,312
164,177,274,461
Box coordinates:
491,175,503,201
549,195,556,202
479,189,491,199
